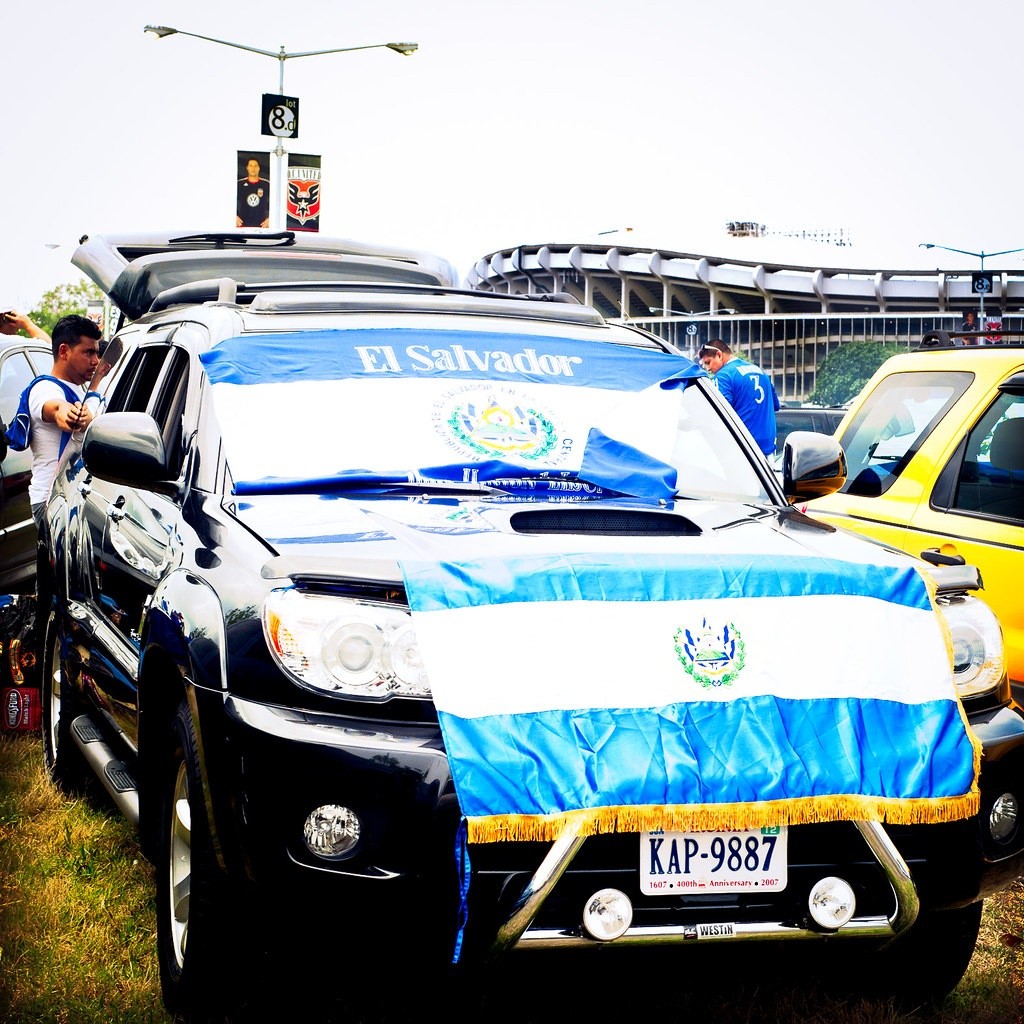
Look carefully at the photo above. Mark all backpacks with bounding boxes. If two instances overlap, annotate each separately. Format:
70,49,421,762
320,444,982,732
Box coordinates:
0,594,42,738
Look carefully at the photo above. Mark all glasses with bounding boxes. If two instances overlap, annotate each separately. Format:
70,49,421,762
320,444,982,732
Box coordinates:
699,343,723,358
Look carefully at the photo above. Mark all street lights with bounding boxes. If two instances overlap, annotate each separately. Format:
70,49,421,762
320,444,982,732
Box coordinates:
918,243,1024,344
140,24,421,227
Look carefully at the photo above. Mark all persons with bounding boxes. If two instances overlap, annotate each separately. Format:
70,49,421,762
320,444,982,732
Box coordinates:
700,338,780,461
0,308,107,659
962,313,976,345
48,336,123,551
236,160,270,230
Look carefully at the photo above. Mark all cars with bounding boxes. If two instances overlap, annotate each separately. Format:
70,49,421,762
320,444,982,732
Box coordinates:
1,224,1024,1024
